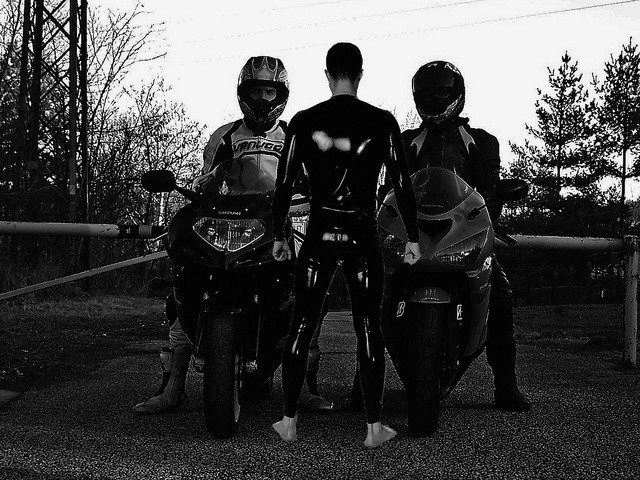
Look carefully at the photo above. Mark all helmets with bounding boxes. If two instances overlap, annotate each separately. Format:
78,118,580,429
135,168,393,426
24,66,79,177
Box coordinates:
237,56,289,123
412,61,464,126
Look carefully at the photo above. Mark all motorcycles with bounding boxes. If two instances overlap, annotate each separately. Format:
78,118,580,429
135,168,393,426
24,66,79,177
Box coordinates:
141,169,312,429
375,168,531,439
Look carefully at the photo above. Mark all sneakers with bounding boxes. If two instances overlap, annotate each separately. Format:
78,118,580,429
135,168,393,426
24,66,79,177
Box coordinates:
300,390,334,412
133,394,185,414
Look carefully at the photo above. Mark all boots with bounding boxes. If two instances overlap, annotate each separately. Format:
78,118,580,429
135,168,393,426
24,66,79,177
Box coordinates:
350,378,363,406
494,374,530,411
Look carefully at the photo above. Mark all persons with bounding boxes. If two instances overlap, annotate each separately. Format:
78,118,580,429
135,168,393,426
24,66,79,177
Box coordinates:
334,59,534,413
132,56,337,417
272,40,423,450
